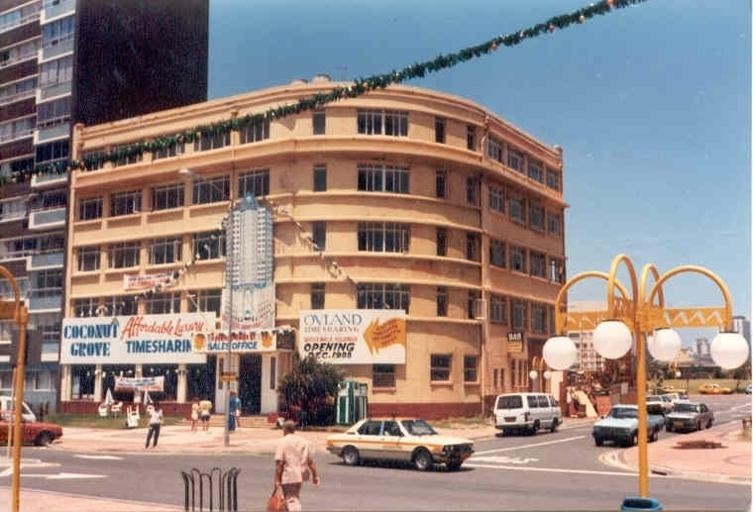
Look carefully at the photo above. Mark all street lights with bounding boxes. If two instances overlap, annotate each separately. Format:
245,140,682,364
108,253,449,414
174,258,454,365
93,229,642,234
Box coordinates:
530,356,551,391
542,254,750,502
178,164,238,446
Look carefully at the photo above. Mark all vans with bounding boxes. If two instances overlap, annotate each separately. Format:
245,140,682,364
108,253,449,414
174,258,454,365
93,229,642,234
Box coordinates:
1,396,36,424
494,391,564,436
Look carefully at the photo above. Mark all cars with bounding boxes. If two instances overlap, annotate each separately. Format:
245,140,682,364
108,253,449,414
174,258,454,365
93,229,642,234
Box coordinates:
0,410,63,447
591,382,713,448
326,415,475,472
698,383,733,396
743,383,752,396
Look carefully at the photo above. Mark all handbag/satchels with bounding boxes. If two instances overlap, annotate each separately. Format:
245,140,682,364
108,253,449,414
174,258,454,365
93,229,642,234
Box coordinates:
267,485,288,512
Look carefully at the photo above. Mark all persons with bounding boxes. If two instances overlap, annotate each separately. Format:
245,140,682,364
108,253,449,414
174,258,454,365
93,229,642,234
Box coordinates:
233,390,241,428
143,400,163,450
271,417,319,511
228,389,235,430
190,396,200,431
197,392,213,431
